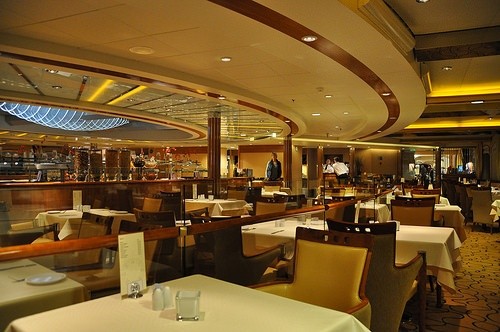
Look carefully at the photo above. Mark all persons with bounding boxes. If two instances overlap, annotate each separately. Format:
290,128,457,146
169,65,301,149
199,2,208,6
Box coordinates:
267,153,282,181
322,159,335,174
427,165,434,183
332,157,350,185
129,156,144,179
420,164,426,186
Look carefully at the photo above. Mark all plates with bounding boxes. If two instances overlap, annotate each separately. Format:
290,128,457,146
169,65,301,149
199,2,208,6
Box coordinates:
48,210,61,213
114,210,128,214
25,273,66,284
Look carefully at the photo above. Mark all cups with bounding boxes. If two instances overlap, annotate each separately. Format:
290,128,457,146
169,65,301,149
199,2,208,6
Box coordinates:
175,289,201,321
208,194,214,201
83,205,91,212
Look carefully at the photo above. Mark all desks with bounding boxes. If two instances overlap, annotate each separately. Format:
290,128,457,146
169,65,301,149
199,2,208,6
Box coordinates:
392,224,463,309
434,205,468,243
36,208,136,241
241,217,329,274
0,260,90,332
255,190,286,214
355,203,391,223
186,199,247,216
5,274,368,332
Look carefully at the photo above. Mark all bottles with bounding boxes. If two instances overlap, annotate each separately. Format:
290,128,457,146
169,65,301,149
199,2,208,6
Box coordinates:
275,219,286,227
153,284,174,310
77,204,83,211
198,192,205,201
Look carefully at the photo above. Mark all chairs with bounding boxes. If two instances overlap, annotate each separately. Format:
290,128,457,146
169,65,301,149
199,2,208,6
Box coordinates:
38,173,500,332
